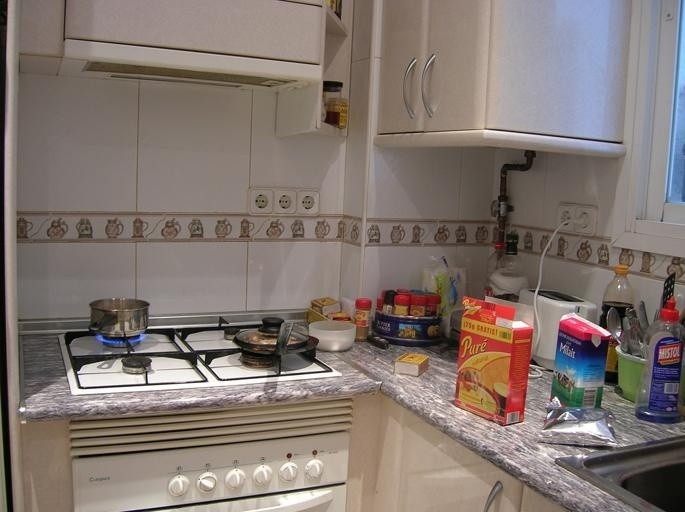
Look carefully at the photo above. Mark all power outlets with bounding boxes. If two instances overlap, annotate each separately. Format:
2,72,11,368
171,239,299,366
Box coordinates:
555,203,597,236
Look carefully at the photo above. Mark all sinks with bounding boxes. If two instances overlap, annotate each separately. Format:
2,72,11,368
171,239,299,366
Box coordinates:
555,434,685,511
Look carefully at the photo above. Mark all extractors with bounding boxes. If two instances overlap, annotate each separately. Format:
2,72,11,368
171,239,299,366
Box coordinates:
48,1,320,92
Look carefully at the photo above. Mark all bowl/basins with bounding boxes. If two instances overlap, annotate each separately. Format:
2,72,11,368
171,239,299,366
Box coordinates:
310,321,356,353
234,317,319,367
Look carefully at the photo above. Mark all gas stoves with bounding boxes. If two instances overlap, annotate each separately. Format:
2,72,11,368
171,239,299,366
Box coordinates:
59,320,353,395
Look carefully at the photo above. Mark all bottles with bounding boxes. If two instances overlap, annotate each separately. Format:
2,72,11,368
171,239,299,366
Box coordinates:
353,289,441,344
599,264,683,421
479,238,524,300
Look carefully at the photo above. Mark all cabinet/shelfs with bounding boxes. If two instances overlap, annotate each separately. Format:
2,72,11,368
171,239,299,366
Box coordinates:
374,0,633,156
275,2,353,138
369,392,568,511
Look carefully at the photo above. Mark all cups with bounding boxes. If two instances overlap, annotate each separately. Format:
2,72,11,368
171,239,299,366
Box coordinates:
324,77,350,129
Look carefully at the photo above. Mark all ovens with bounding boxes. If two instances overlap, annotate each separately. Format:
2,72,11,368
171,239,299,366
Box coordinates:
71,433,350,512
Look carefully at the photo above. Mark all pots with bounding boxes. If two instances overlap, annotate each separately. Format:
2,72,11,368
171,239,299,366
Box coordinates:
88,298,151,338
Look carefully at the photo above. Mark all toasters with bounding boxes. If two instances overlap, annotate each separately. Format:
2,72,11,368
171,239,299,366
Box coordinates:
519,288,598,373
